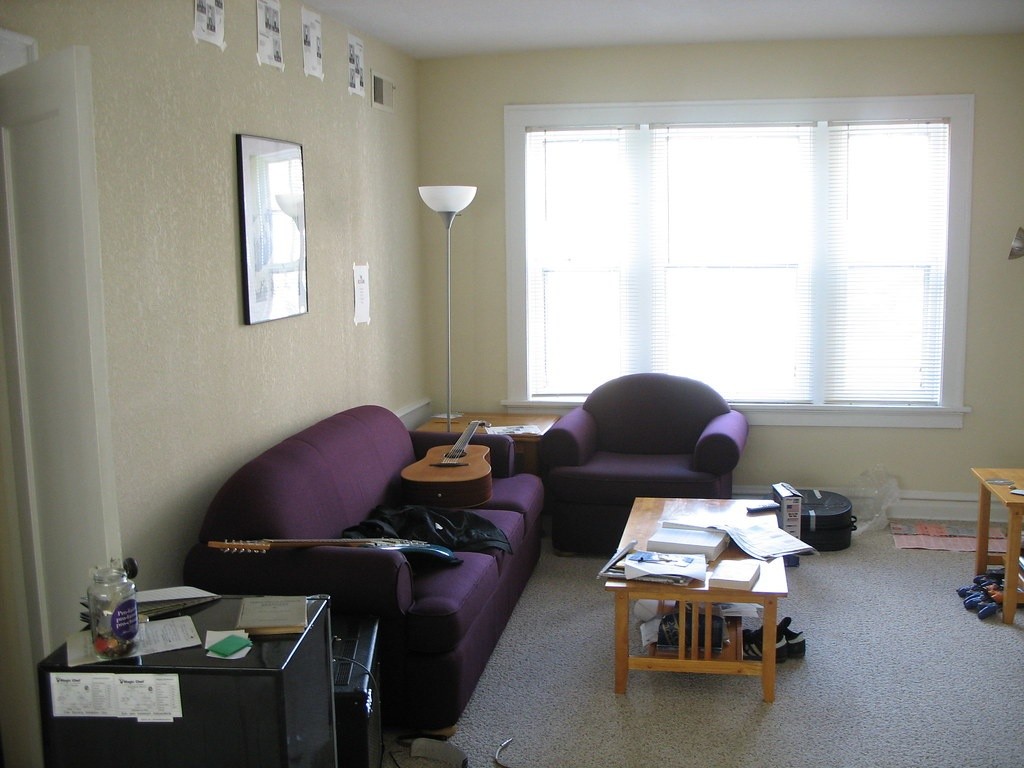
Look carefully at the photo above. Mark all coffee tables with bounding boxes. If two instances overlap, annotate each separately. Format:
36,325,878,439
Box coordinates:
602,497,788,703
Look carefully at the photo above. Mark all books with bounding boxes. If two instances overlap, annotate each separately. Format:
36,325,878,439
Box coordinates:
656,611,724,653
234,596,308,634
646,516,760,591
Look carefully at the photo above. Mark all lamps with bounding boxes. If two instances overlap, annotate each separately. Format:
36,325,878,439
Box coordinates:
418,181,476,442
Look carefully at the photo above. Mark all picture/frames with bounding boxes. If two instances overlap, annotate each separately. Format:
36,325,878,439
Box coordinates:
237,133,309,325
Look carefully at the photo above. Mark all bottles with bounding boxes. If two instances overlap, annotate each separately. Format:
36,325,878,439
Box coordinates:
88,568,141,659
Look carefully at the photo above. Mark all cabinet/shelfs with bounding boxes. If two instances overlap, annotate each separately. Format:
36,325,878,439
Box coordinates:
36,594,338,768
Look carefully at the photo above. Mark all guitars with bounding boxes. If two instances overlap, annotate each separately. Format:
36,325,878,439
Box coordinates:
206,536,457,558
400,418,493,509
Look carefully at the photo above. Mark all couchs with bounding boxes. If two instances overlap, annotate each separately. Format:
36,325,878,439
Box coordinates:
185,405,545,730
536,375,748,555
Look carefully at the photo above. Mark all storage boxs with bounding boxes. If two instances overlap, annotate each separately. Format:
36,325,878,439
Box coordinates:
772,482,804,567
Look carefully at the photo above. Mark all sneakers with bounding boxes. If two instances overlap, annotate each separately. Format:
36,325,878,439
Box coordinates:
740,628,788,663
759,616,807,657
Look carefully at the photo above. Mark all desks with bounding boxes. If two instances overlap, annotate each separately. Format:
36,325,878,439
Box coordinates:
968,464,1024,623
410,412,561,477
331,618,382,768
